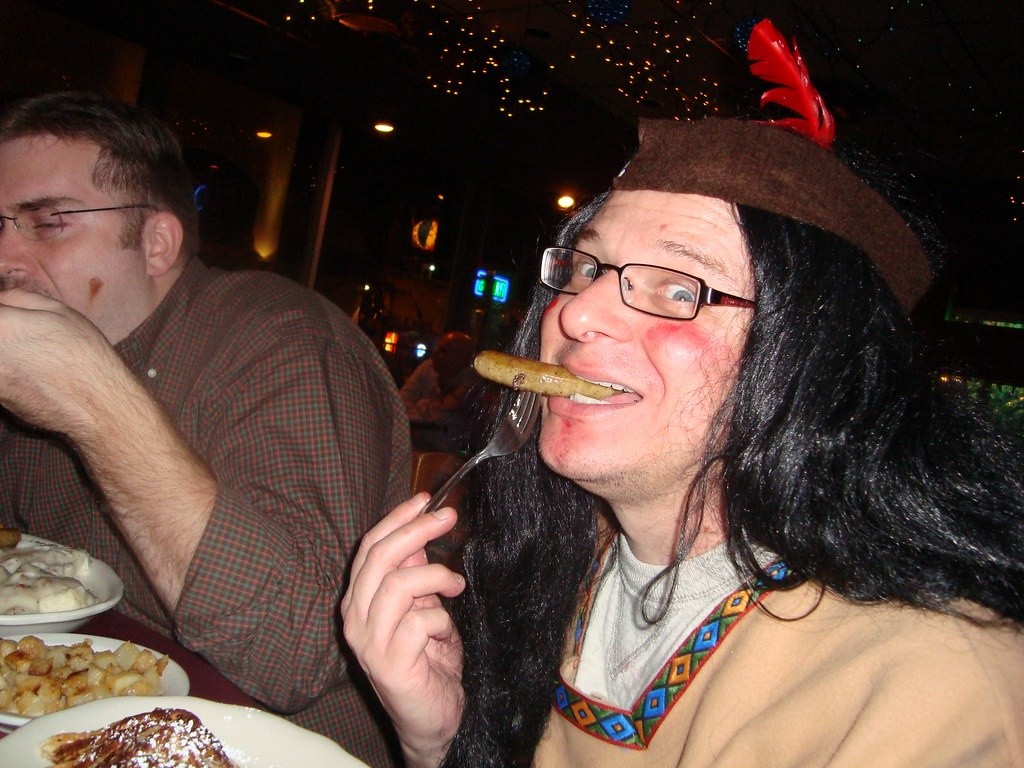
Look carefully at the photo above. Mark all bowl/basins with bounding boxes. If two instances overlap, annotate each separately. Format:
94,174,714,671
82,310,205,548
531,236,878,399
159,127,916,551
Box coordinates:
0,529,125,637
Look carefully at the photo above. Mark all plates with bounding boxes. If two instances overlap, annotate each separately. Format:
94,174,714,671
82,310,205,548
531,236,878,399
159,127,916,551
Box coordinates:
1,697,374,768
0,634,189,733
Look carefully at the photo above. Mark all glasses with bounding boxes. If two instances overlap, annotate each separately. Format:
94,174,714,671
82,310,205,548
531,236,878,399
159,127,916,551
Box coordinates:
539,246,754,320
0,204,160,241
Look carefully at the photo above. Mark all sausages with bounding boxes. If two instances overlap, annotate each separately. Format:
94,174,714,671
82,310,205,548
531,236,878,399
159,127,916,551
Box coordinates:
474,351,624,398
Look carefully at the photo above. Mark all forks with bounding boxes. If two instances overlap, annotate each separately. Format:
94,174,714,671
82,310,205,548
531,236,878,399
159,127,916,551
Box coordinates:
416,390,542,516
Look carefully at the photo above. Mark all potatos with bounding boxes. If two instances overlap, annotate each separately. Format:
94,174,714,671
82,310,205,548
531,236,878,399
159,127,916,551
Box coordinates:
1,636,168,717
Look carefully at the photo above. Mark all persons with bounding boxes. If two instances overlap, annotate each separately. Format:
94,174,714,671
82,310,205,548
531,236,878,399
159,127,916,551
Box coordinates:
341,118,1024,768
398,332,475,426
1,93,410,768
